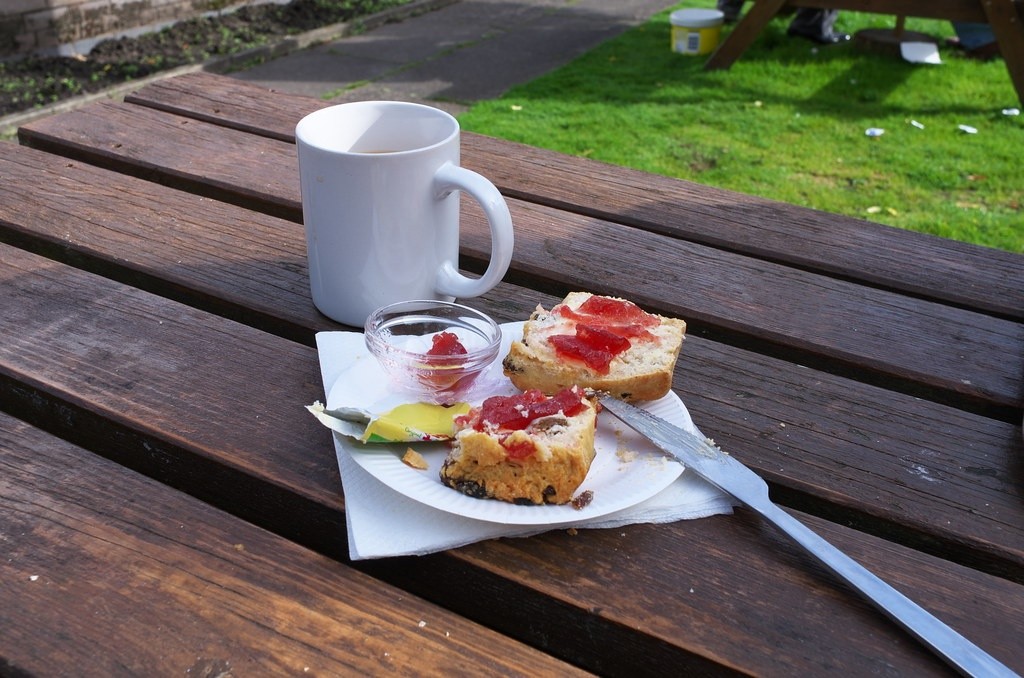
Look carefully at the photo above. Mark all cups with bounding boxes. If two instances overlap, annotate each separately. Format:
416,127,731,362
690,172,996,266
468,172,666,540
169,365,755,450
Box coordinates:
295,99,517,318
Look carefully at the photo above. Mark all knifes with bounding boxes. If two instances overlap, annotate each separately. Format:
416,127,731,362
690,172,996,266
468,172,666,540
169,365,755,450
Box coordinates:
597,386,1022,678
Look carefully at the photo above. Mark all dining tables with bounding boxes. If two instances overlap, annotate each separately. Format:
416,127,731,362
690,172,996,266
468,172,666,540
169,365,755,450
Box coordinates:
1,69,1023,678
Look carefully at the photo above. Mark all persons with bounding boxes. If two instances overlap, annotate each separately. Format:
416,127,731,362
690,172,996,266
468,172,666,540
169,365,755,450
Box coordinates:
704,0,1024,62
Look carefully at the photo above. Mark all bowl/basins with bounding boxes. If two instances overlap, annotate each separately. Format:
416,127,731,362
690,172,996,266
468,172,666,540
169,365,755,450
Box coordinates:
363,294,505,406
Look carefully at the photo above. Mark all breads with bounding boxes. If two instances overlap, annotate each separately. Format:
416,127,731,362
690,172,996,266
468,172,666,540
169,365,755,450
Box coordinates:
438,289,689,509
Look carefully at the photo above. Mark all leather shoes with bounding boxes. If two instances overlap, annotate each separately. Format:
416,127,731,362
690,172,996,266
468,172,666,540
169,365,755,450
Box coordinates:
786,27,851,45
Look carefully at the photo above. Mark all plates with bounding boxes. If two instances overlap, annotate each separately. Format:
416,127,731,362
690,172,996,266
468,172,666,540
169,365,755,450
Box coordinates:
325,331,693,525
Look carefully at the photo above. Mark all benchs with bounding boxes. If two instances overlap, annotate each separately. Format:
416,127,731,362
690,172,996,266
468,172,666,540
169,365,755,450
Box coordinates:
703,0,1024,108
